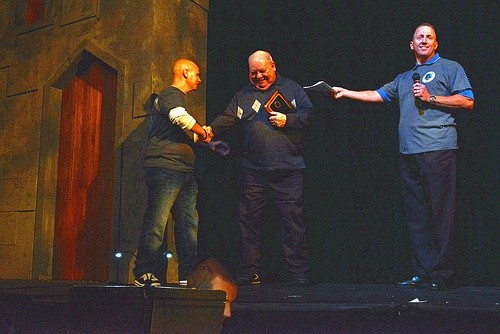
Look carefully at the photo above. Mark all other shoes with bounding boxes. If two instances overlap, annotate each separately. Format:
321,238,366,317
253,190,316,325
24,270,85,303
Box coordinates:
431,280,440,290
239,273,261,285
396,276,424,286
294,276,307,287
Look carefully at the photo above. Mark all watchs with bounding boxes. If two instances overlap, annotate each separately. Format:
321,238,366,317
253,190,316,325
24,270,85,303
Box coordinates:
429,95,436,105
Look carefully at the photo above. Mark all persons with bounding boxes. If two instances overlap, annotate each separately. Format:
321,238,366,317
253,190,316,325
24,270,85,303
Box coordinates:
333,23,474,291
133,59,213,288
186,257,238,321
204,50,315,287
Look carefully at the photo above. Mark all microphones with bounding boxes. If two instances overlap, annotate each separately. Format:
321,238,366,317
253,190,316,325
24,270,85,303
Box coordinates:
412,73,422,108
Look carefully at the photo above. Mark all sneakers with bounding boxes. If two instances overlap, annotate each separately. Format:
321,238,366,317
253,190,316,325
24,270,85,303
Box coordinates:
134,273,161,286
179,279,188,286
